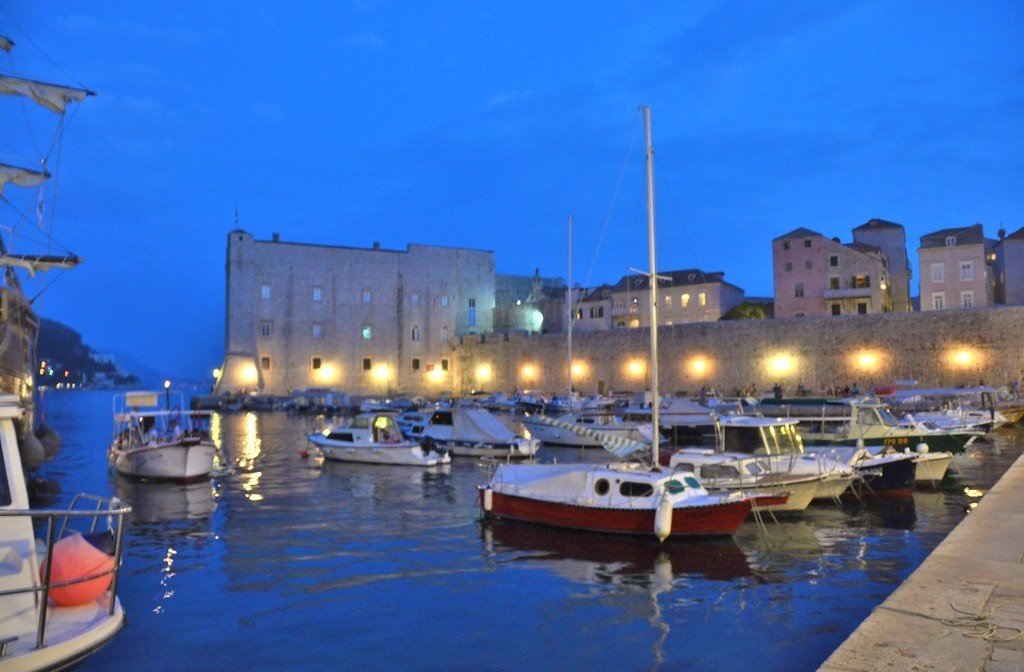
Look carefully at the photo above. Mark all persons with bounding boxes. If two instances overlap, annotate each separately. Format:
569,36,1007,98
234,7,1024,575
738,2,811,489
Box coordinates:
698,378,1022,411
113,405,160,450
168,418,185,441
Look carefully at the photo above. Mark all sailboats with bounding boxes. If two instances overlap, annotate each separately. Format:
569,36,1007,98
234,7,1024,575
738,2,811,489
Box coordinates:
0,26,132,672
474,101,792,539
494,214,667,449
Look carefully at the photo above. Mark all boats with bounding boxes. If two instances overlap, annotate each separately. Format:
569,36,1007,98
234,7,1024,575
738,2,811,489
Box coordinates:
632,391,1024,513
397,406,541,457
308,411,451,466
361,398,622,414
108,381,214,479
